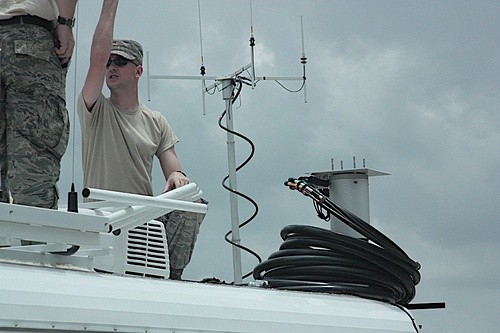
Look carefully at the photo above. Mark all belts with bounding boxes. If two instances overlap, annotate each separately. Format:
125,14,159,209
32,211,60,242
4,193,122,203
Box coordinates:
2,14,54,31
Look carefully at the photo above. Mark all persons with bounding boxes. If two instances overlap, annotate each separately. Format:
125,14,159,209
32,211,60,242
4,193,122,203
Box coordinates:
76,0,208,280
0,0,79,256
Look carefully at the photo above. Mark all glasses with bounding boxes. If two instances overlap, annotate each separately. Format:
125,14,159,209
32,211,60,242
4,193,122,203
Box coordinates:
105,56,138,70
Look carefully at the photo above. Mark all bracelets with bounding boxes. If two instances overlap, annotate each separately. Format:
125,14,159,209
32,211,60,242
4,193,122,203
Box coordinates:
177,170,186,176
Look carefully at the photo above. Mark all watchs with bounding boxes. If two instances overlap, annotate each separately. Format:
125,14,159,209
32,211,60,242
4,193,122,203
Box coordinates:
58,15,75,27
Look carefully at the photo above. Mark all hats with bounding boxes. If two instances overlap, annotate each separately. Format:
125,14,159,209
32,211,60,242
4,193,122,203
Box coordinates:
112,40,144,64
